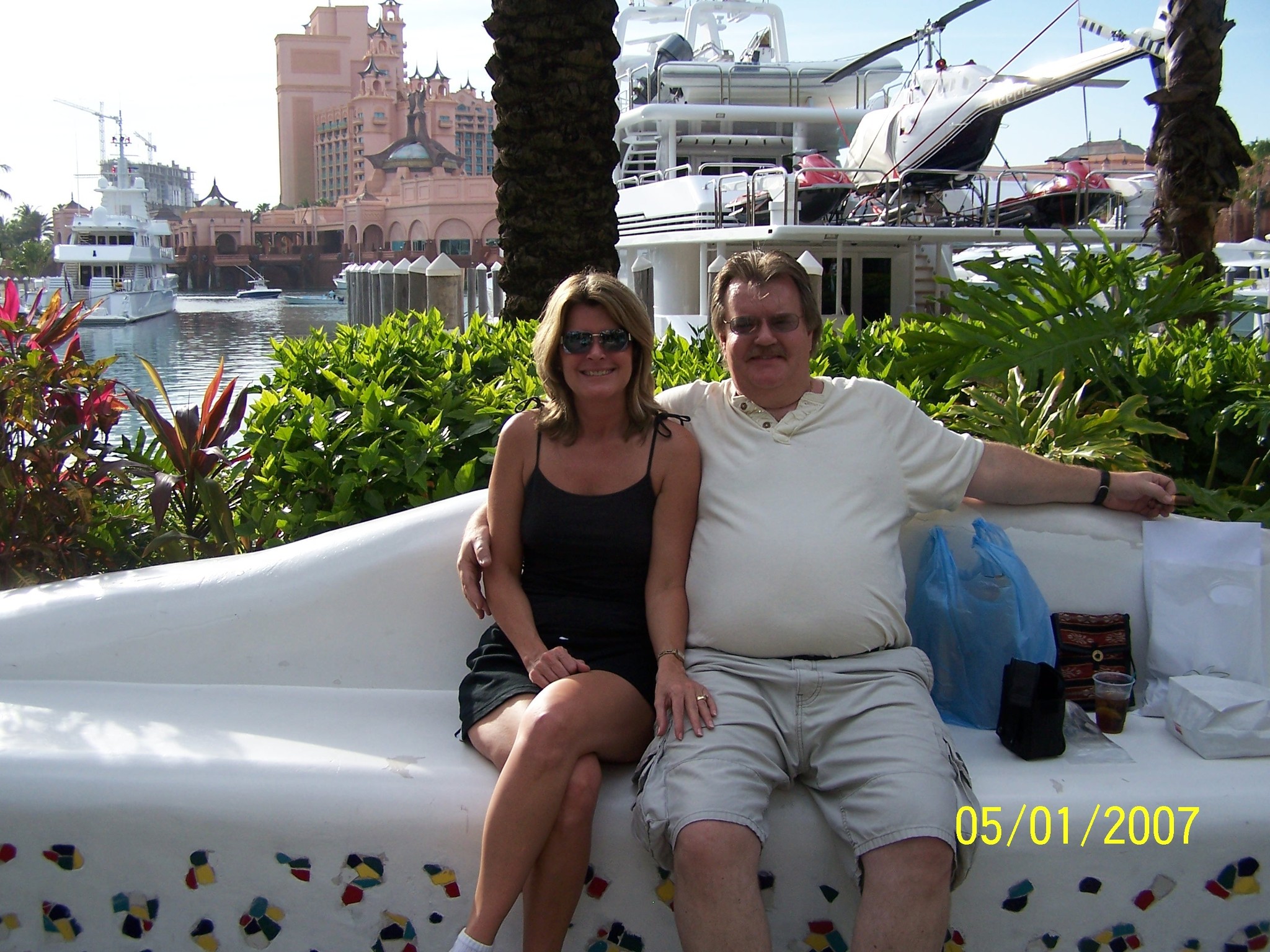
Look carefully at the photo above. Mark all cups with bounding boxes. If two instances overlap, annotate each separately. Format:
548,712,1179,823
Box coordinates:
1092,671,1136,734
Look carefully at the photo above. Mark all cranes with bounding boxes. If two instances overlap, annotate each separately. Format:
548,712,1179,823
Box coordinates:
59,99,156,172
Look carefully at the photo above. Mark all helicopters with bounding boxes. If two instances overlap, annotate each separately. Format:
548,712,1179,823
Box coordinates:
824,1,1167,182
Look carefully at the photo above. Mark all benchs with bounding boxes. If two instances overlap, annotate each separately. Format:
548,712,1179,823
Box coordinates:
0,488,1270,952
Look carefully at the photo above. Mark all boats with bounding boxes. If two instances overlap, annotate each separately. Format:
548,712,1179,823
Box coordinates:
614,0,1174,342
236,277,282,299
283,295,345,303
27,125,179,325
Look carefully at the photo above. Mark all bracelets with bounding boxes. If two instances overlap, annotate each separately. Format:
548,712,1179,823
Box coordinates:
1091,463,1112,507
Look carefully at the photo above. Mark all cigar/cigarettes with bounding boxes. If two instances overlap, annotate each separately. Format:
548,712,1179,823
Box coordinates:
1169,495,1194,507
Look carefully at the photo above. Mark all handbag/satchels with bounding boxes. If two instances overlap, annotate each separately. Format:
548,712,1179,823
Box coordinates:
1051,611,1137,710
908,516,1056,730
995,658,1066,761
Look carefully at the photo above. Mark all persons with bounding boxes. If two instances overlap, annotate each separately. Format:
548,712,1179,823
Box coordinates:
457,251,1178,952
328,289,336,300
446,275,718,952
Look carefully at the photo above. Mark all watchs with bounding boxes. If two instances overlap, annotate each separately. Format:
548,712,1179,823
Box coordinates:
657,648,686,663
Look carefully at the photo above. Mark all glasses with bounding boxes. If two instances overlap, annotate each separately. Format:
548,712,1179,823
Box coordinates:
559,328,635,354
723,312,808,335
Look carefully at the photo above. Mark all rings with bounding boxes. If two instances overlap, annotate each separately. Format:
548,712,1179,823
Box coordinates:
696,694,709,702
